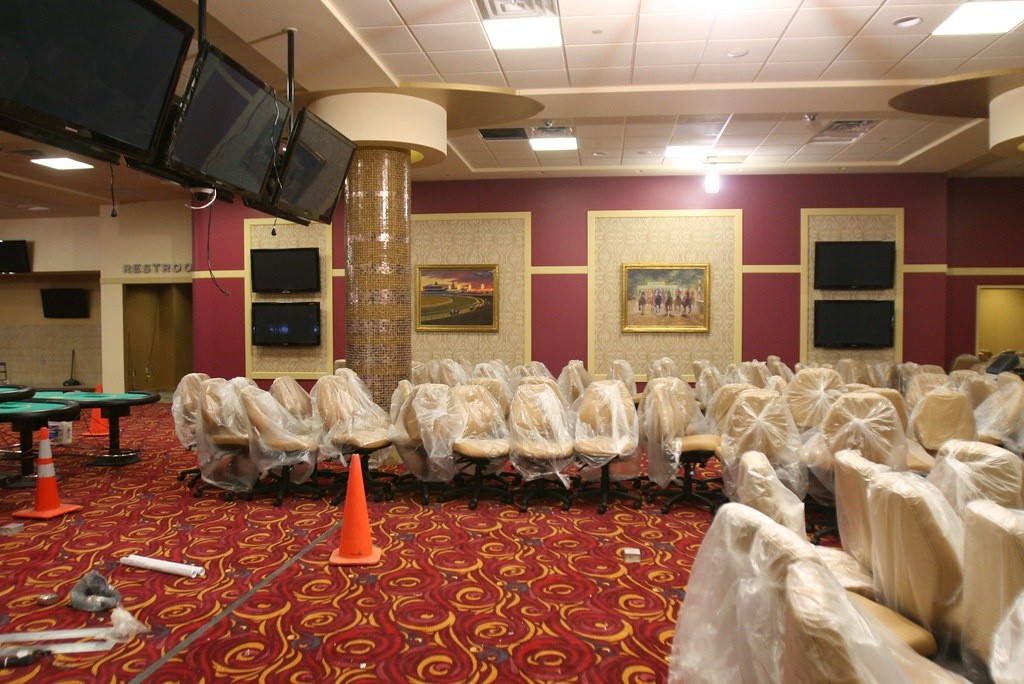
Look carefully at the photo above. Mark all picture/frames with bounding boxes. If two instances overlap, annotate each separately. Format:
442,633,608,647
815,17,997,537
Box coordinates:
621,263,710,333
413,264,499,334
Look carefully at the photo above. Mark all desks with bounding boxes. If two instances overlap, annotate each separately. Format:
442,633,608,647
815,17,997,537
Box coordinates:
26,390,161,468
0,384,39,460
33,383,96,392
0,398,82,490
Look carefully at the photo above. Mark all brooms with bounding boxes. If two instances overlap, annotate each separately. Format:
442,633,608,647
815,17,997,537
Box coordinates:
63,349,81,386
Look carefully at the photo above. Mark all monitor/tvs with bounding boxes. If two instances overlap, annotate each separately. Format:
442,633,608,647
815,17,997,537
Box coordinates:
251,302,320,346
813,240,895,290
0,239,30,273
0,0,357,226
814,300,895,347
250,248,321,293
40,288,89,319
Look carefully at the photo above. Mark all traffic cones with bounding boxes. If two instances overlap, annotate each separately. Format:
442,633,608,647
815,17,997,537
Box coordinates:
80,384,110,436
328,453,382,567
12,427,81,520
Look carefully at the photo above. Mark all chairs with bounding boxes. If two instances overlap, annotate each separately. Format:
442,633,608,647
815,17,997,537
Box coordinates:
389,379,464,487
240,385,324,508
333,367,398,481
270,377,334,481
441,384,514,510
671,439,1024,684
510,376,581,491
409,356,1024,436
501,384,574,512
723,389,819,535
392,382,461,505
803,392,909,546
309,375,394,507
464,377,522,489
570,379,643,515
170,371,212,488
638,377,723,518
191,378,272,501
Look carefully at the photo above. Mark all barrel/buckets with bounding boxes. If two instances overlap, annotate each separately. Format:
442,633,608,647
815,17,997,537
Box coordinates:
48,421,72,445
